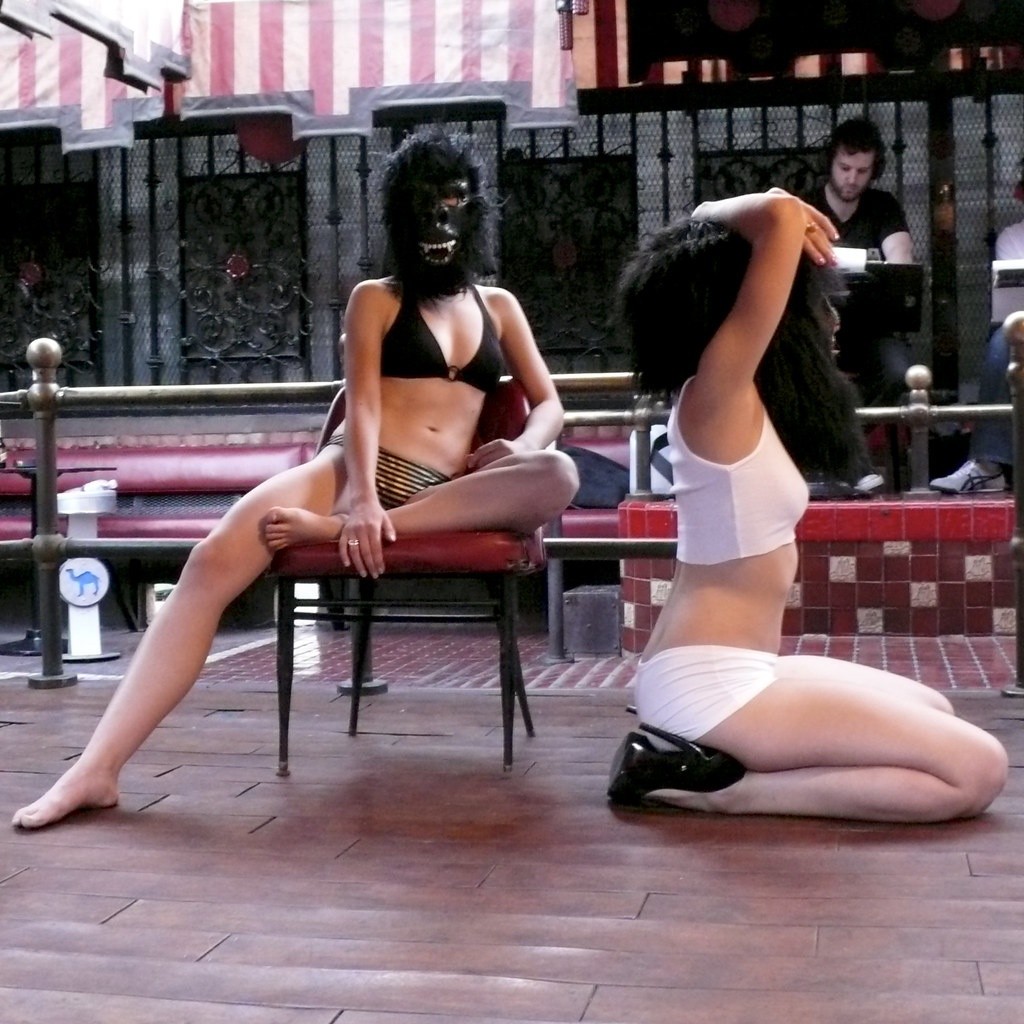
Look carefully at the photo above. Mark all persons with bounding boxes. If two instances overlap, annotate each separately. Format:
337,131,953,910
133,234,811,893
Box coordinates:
928,157,1024,493
605,185,1010,823
795,117,915,499
10,126,581,833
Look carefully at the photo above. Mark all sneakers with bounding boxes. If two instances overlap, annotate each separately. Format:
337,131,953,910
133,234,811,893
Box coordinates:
929,458,1010,493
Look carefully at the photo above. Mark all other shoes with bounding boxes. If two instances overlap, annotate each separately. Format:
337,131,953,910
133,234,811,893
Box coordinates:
838,472,883,501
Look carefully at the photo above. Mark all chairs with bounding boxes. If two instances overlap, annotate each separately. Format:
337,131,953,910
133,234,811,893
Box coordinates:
260,374,544,777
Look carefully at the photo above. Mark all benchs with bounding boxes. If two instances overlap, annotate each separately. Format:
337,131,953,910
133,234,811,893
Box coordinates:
0,425,631,632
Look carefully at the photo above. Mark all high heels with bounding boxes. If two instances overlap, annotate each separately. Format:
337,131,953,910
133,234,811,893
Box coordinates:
606,704,748,809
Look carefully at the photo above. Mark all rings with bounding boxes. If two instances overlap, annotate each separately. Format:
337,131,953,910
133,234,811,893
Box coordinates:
345,540,360,546
806,221,822,234
